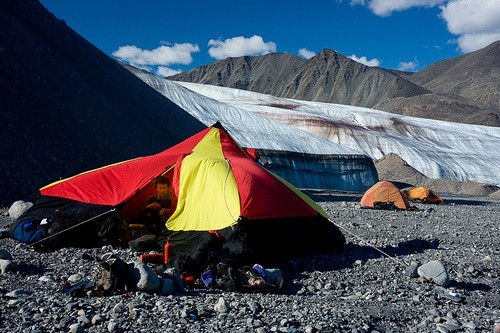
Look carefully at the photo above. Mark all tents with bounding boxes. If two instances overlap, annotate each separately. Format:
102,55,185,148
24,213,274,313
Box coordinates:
38,120,346,262
407,187,443,205
361,181,410,210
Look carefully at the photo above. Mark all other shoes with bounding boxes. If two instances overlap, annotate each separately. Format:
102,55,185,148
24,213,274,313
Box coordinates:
94,253,116,291
102,257,128,295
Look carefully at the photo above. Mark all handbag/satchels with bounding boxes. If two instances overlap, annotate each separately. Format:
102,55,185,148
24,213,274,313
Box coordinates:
8,215,67,250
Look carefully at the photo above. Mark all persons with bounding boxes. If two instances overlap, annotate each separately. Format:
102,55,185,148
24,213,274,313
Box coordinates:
144,176,174,235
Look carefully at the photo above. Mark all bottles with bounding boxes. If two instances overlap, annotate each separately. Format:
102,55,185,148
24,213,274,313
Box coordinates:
140,254,164,263
164,237,172,264
